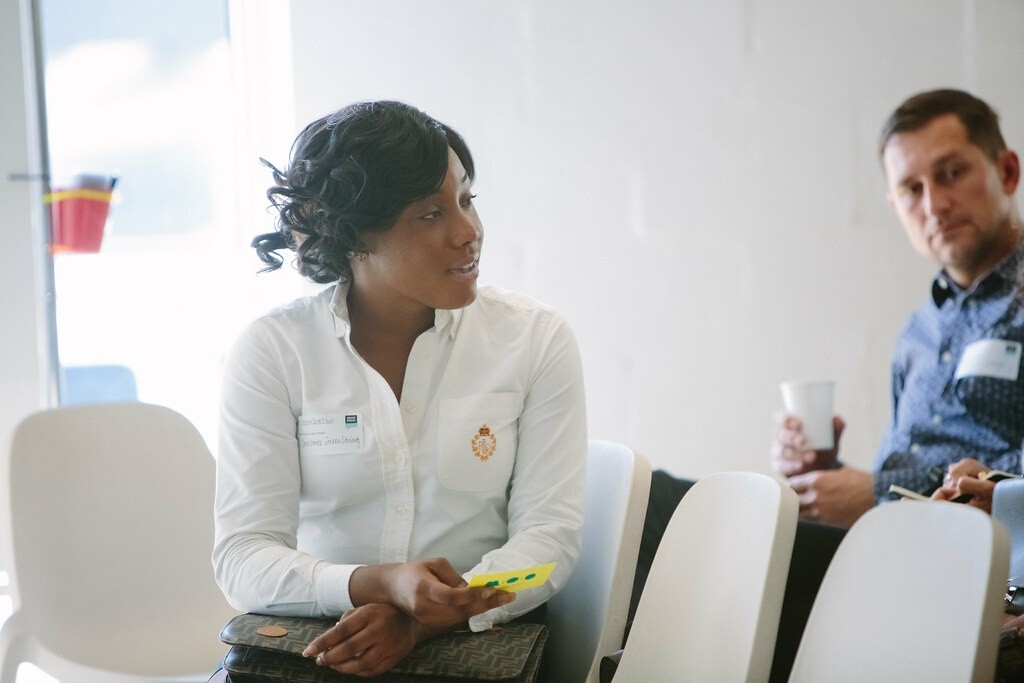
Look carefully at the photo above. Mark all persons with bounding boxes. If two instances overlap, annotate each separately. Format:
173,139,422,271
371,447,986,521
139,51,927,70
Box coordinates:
773,89,1024,683
213,101,588,683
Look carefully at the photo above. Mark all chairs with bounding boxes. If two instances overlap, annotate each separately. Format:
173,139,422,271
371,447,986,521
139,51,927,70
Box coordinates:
547,439,1014,683
0,401,234,683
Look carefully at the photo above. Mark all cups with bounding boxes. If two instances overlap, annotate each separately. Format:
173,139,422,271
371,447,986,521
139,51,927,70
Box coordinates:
782,379,835,449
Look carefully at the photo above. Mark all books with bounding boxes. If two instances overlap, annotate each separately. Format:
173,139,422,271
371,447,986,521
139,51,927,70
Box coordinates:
888,469,1020,505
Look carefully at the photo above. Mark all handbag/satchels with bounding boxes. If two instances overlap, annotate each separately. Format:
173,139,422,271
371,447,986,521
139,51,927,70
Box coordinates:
218,614,549,683
